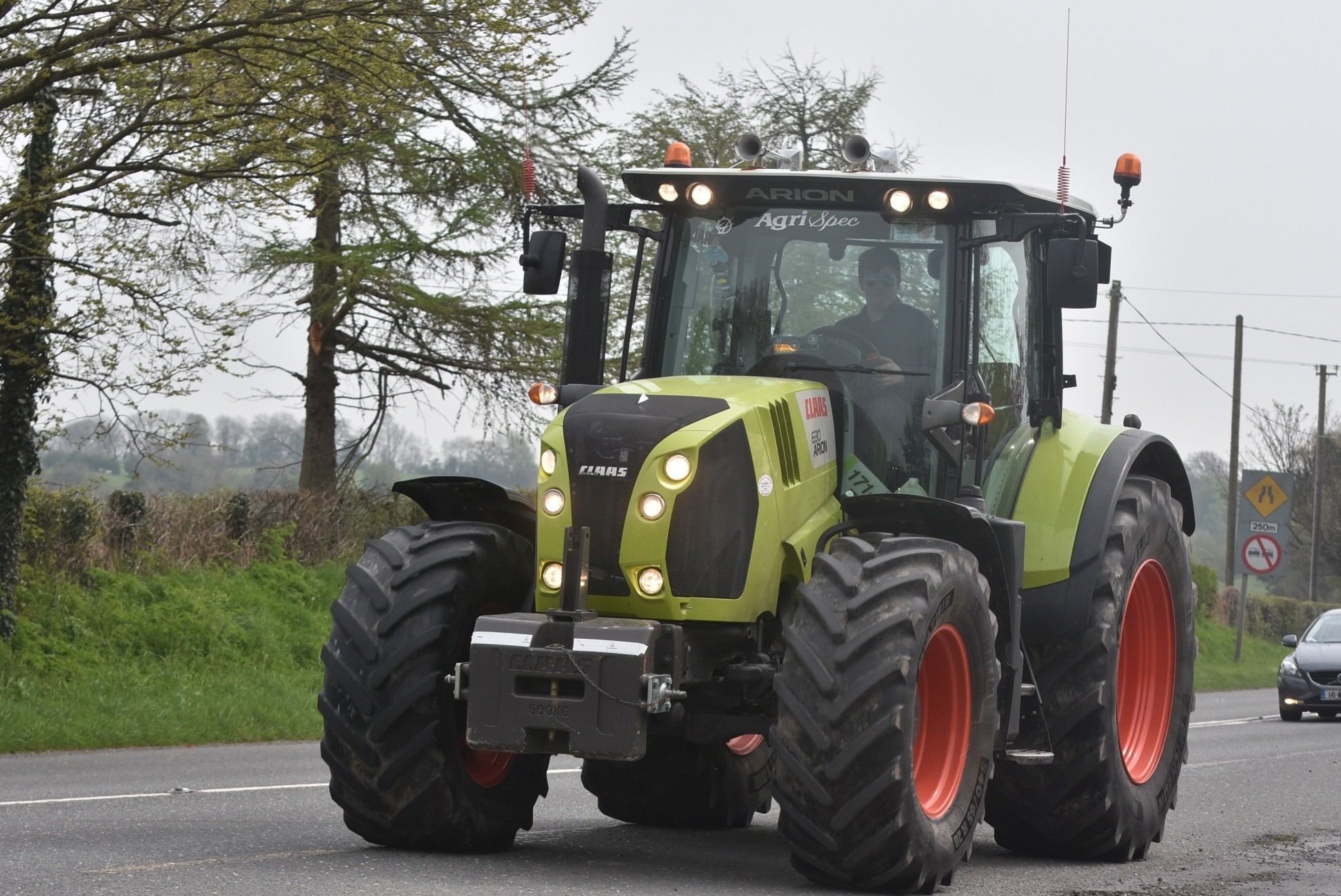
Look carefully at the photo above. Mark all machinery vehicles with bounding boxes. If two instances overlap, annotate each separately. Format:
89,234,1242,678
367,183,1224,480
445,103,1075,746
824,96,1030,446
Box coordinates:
314,128,1200,896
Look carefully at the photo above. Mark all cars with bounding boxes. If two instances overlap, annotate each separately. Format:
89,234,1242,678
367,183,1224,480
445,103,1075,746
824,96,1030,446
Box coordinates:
1276,609,1341,722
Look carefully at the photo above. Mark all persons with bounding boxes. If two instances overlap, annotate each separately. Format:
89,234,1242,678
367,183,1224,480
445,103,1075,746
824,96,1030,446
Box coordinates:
834,246,937,413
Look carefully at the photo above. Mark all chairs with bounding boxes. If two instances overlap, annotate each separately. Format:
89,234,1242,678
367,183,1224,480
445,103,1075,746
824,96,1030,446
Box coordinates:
963,361,1021,461
